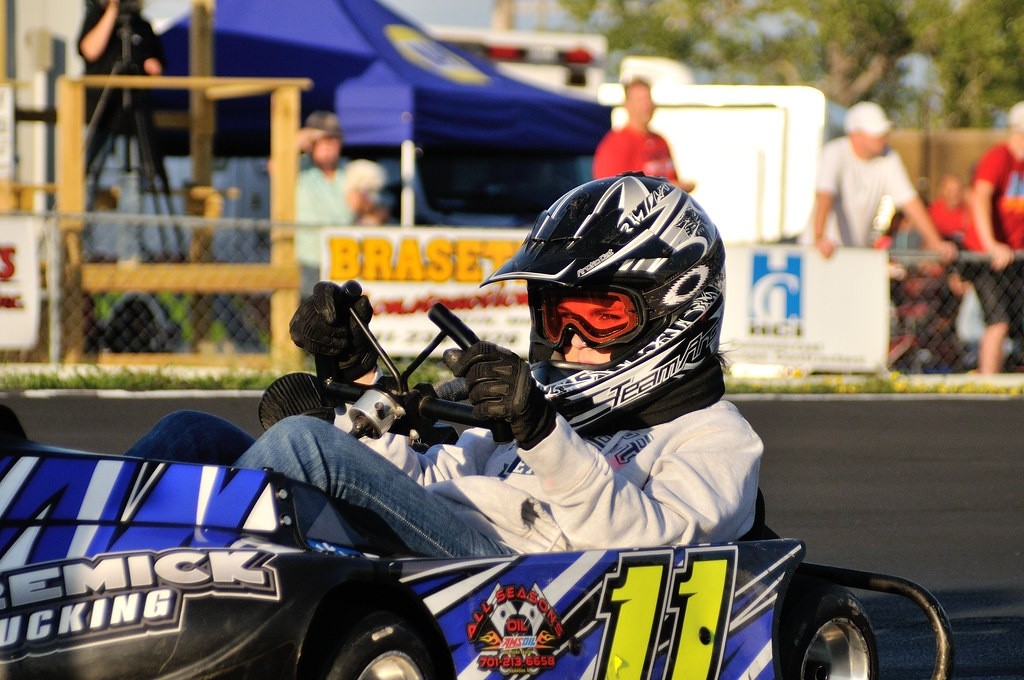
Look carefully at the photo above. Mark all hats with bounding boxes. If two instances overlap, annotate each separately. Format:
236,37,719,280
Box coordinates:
1008,102,1024,131
844,101,896,138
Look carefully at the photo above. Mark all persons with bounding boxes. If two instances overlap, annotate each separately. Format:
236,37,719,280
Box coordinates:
86,191,141,262
974,101,1024,373
799,101,956,262
591,78,694,192
121,172,764,559
295,112,390,226
928,176,984,252
78,1,164,131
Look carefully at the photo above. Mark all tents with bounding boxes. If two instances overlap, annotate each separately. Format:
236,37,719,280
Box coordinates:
143,0,614,228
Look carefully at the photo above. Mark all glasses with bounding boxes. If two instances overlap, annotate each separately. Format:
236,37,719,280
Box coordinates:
539,287,641,348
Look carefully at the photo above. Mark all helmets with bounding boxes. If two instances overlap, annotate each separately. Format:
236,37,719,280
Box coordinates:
339,157,387,217
305,110,343,169
480,171,726,440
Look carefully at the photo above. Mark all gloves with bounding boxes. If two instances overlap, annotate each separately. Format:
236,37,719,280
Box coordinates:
443,341,556,450
290,281,379,382
